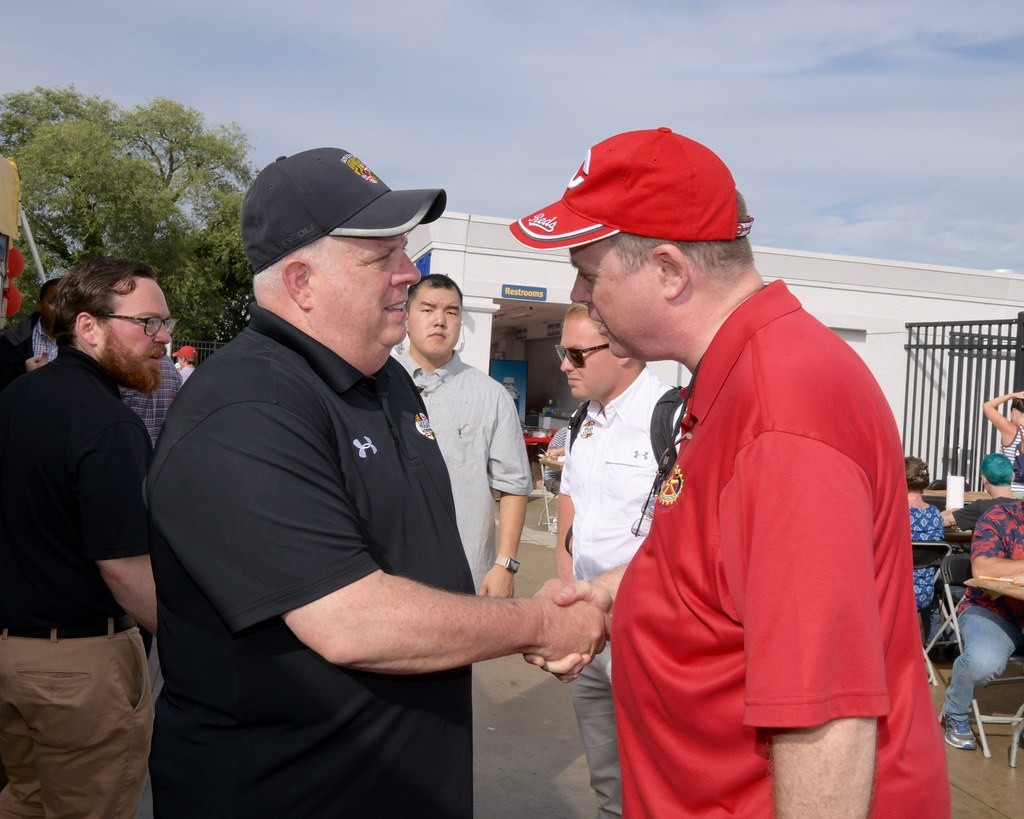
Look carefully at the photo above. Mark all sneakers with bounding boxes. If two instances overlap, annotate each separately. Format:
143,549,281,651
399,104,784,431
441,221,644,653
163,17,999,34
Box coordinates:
939,710,977,749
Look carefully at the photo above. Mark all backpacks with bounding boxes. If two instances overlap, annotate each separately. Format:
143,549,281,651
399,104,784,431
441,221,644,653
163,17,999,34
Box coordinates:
1013,425,1024,482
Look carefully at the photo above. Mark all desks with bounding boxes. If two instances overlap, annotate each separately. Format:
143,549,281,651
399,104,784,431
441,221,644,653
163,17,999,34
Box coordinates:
523,434,553,465
922,489,994,501
964,577,1024,767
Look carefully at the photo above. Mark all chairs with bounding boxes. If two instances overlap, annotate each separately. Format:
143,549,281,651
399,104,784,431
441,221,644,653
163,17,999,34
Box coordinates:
539,441,558,532
912,481,1024,758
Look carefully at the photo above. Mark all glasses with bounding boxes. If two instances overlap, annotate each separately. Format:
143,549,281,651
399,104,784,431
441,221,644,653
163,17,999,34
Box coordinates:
555,344,609,367
90,314,179,336
631,476,659,536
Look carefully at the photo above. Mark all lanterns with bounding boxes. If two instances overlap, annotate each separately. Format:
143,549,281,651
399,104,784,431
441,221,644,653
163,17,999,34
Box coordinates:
6,284,22,317
8,246,24,280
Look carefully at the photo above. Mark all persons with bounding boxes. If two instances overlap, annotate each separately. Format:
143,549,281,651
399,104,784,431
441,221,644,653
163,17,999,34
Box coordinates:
544,426,569,533
507,128,953,819
556,304,687,819
982,391,1024,484
898,452,1024,750
396,273,534,602
0,254,182,819
172,345,198,385
142,147,609,819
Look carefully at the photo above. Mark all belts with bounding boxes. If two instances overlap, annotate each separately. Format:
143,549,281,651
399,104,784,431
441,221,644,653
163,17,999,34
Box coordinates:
0,615,136,638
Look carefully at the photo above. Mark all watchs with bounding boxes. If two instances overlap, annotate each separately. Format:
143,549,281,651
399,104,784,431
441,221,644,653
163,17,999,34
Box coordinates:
496,556,521,574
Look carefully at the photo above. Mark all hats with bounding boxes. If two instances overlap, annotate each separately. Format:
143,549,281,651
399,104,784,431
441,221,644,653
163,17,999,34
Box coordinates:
240,148,447,275
172,346,198,359
509,128,754,251
